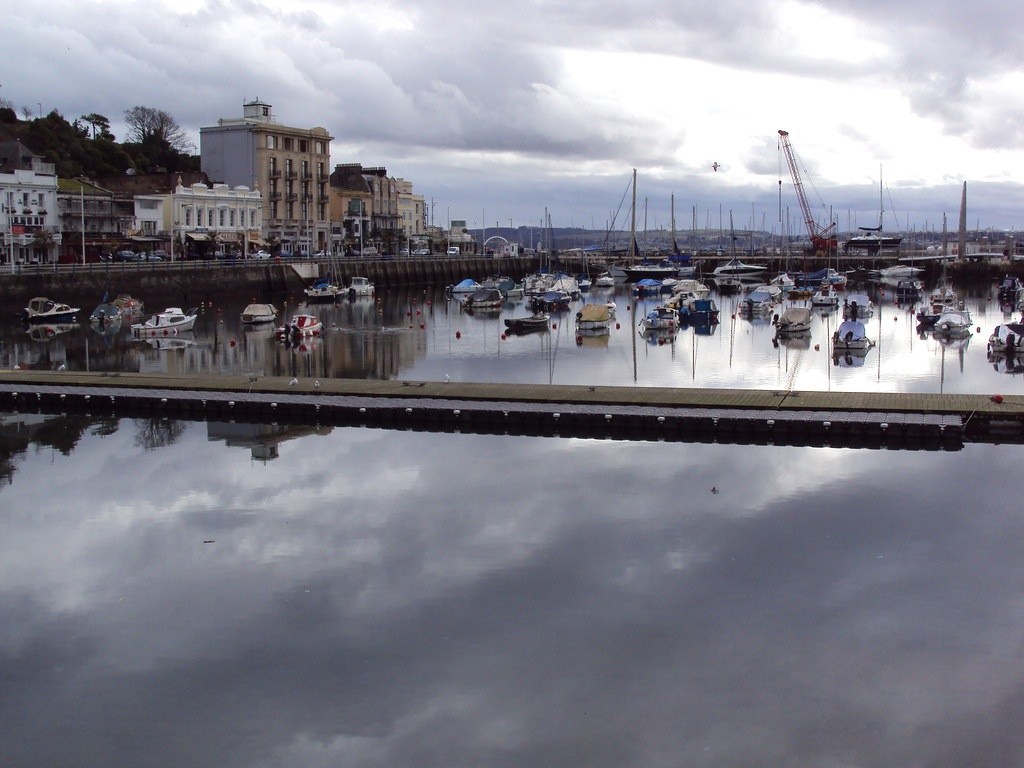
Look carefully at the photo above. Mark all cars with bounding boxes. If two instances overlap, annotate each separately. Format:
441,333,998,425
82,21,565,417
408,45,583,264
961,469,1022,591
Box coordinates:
400,247,431,256
236,250,272,259
313,249,329,257
117,251,167,262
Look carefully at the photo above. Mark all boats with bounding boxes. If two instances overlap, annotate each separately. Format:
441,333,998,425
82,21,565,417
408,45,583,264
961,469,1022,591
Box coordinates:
239,303,279,324
575,300,616,330
643,298,720,329
833,292,874,349
446,273,571,311
504,313,550,332
348,276,375,296
737,285,814,331
90,291,199,335
20,297,80,322
917,276,1024,352
275,314,322,338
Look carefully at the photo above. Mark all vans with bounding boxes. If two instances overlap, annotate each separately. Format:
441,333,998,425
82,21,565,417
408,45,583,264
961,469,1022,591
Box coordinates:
447,247,459,254
363,247,377,255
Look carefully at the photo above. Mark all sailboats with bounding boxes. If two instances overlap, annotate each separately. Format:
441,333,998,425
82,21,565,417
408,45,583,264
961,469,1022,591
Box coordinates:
304,217,349,302
521,163,957,302
